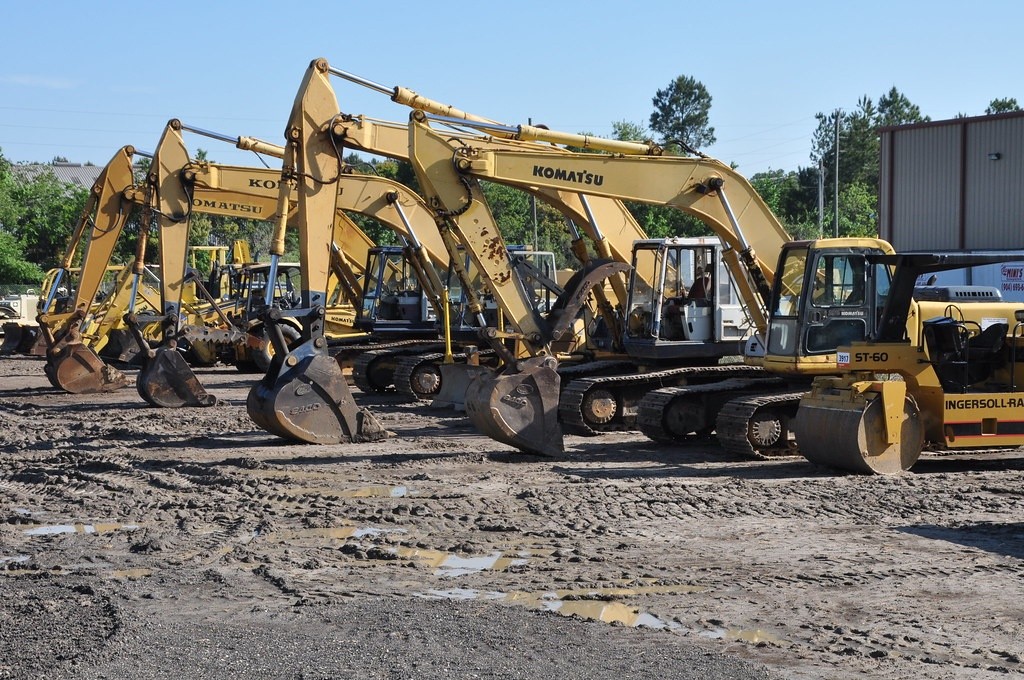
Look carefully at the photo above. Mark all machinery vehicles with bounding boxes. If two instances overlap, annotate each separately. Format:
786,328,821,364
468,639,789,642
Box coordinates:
1,56,1023,476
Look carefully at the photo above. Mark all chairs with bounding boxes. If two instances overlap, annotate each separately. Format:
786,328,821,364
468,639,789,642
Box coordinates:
827,273,862,348
969,323,1010,387
662,265,713,340
